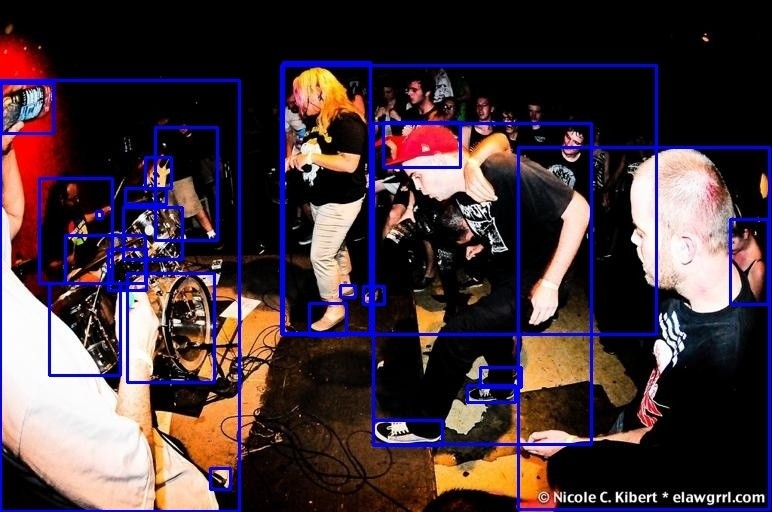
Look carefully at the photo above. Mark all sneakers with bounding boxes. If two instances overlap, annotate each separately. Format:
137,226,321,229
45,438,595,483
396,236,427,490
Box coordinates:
211,468,230,490
363,287,385,304
373,421,442,445
341,285,355,298
467,385,515,403
481,367,521,387
310,303,347,336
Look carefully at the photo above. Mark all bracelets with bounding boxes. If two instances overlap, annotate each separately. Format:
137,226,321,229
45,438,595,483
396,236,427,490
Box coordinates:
96,211,103,218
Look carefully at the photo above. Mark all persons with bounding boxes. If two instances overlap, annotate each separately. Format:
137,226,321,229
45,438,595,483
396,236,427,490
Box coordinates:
730,220,769,304
519,147,770,509
373,124,592,445
282,66,656,335
39,179,113,283
156,128,217,240
0,81,240,511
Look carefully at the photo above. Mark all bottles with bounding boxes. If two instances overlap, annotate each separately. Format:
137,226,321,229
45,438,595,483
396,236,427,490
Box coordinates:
1,83,53,133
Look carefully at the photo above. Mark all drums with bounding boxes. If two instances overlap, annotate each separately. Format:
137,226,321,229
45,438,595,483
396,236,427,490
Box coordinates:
124,208,182,260
109,250,146,291
128,273,215,383
50,284,121,375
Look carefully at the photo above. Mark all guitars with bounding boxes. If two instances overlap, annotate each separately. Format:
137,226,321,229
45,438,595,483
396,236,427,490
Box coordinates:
145,157,172,188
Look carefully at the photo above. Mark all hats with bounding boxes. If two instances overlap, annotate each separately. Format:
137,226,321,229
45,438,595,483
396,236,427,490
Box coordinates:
384,123,461,168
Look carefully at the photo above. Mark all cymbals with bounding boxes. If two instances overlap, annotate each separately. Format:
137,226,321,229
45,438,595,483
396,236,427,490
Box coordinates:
66,236,146,284
125,188,166,205
